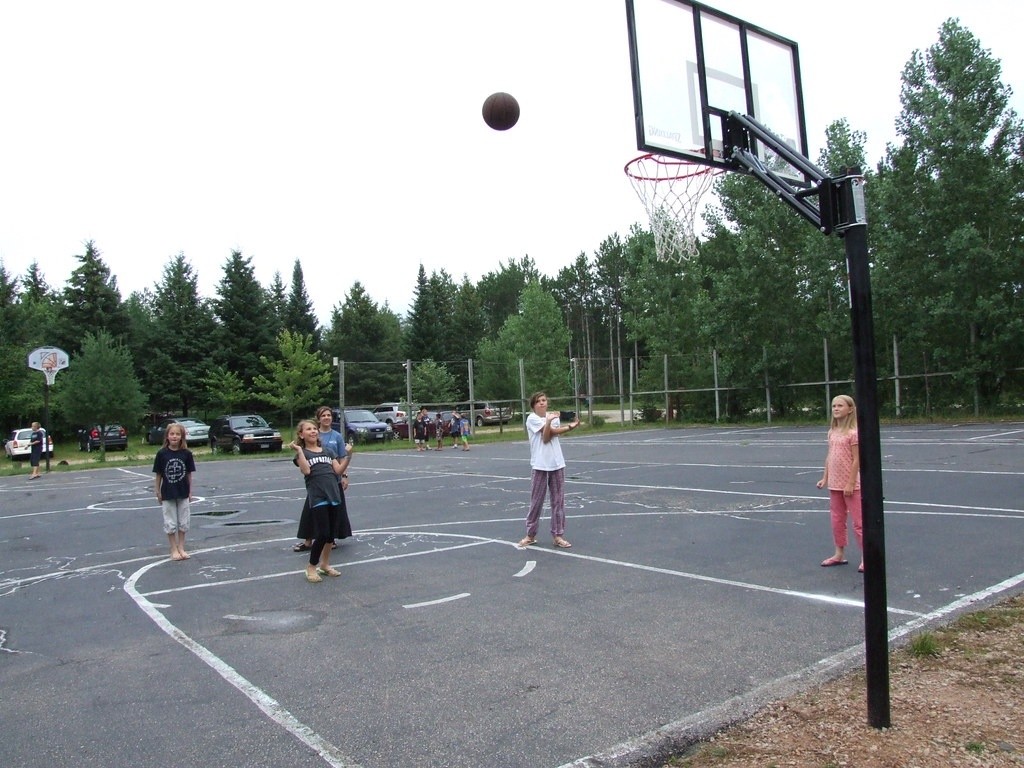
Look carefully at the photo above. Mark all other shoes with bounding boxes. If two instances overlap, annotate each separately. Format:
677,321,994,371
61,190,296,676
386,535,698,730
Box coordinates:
170,551,190,561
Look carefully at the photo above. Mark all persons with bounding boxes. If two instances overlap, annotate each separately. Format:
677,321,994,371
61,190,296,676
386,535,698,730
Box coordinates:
518,391,580,547
421,409,432,450
460,413,471,450
293,404,348,551
152,423,196,561
24,422,43,479
413,412,427,452
290,418,353,583
816,394,865,573
448,410,461,449
432,414,444,451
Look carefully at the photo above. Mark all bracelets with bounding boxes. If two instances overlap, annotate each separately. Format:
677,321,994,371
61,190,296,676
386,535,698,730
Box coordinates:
341,473,348,478
156,492,161,494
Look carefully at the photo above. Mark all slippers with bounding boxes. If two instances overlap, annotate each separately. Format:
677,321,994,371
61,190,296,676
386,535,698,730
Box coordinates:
305,566,322,583
519,539,537,548
319,569,341,576
331,542,337,549
553,539,572,548
822,559,848,566
294,543,312,552
858,562,866,572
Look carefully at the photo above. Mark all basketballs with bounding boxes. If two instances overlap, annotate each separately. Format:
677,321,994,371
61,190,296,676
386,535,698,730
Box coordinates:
481,92,520,131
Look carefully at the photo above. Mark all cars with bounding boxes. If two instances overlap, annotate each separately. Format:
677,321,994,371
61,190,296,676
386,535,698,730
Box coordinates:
3,426,54,463
393,411,465,438
146,415,211,448
77,423,129,455
455,399,513,426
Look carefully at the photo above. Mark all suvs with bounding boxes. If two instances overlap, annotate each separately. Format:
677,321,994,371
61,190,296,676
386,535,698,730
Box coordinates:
208,413,284,457
371,403,423,430
326,408,393,445
